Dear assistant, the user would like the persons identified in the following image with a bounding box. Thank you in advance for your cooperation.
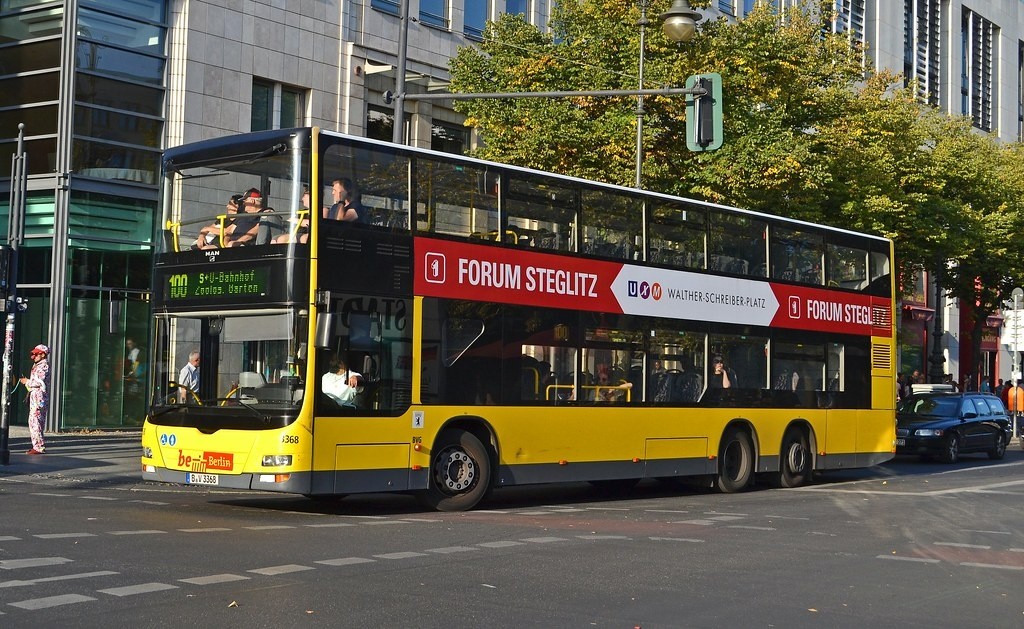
[520,351,734,404]
[895,370,1024,438]
[179,351,202,404]
[322,349,365,408]
[19,344,52,455]
[186,177,361,251]
[122,338,148,426]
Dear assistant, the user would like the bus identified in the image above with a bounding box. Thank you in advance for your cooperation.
[107,125,899,507]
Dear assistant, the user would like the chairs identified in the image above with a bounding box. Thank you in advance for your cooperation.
[551,365,839,403]
[350,351,372,375]
[206,206,822,284]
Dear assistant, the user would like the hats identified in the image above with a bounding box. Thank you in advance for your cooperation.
[238,188,261,200]
[31,344,49,354]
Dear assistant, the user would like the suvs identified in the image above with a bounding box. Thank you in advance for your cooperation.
[891,390,1014,463]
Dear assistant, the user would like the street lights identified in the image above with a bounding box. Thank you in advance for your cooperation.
[633,1,703,401]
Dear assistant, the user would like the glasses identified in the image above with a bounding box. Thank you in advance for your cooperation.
[713,359,723,363]
[32,352,42,356]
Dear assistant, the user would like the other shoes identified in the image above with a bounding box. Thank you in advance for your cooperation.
[26,450,41,454]
